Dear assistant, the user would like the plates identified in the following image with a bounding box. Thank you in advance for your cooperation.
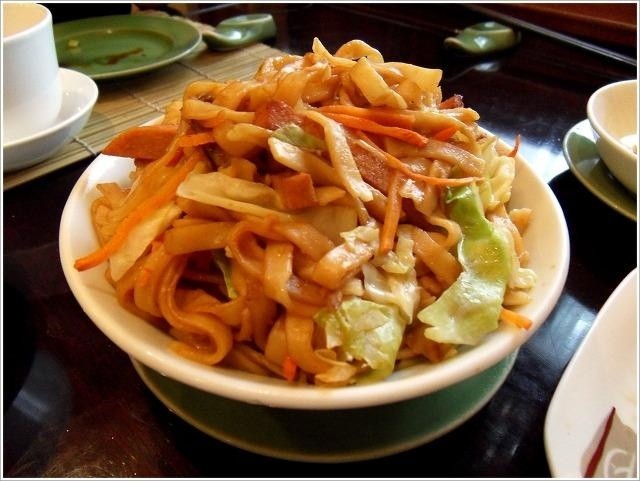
[128,344,523,461]
[562,118,637,224]
[544,265,637,478]
[53,15,203,78]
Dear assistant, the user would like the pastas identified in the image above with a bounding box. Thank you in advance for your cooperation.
[92,38,535,385]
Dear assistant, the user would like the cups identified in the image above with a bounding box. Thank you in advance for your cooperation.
[1,2,61,143]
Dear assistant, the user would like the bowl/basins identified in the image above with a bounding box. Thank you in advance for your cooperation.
[3,67,99,172]
[587,79,637,197]
[59,105,573,410]
